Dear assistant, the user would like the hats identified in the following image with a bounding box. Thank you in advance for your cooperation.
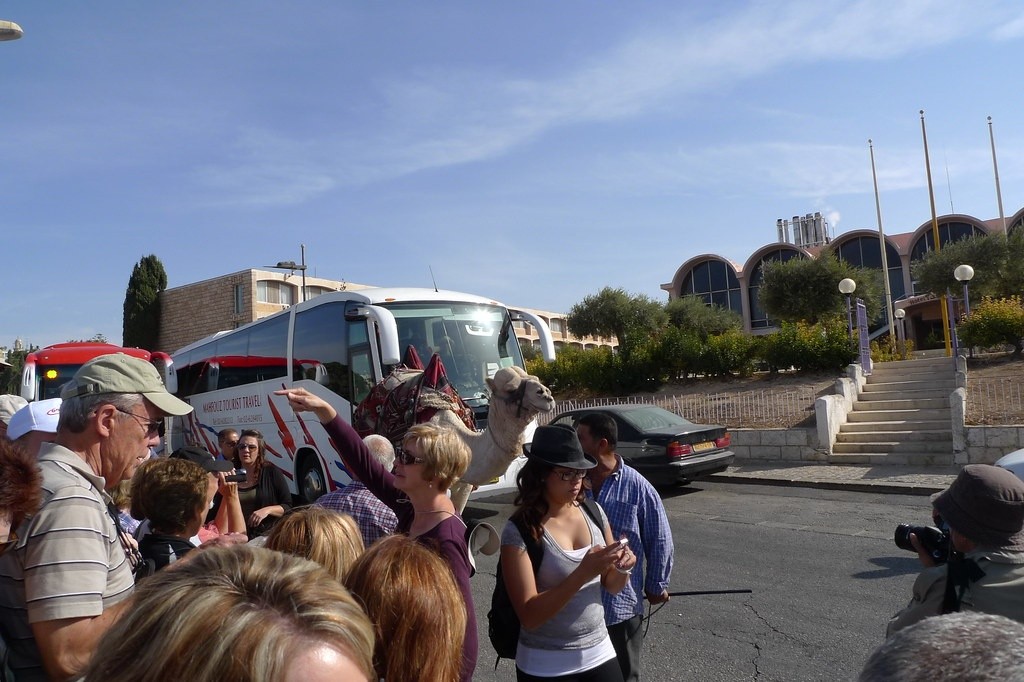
[0,394,29,425]
[7,398,62,441]
[523,423,598,469]
[465,519,501,578]
[61,353,194,417]
[931,464,1024,553]
[169,446,234,473]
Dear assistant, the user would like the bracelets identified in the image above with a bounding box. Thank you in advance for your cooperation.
[615,567,633,575]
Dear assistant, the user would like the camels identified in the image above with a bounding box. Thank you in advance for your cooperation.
[356,366,556,517]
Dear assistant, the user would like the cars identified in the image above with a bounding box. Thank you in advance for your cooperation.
[547,404,736,489]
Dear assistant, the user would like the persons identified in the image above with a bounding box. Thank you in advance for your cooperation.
[6,398,63,458]
[859,610,1024,682]
[108,447,248,575]
[501,424,636,682]
[0,394,29,425]
[886,464,1024,639]
[344,533,463,682]
[439,336,454,355]
[264,507,366,583]
[575,414,674,682]
[314,434,398,547]
[216,429,239,460]
[205,429,292,538]
[0,352,193,682]
[76,543,378,682]
[0,438,46,542]
[273,387,478,682]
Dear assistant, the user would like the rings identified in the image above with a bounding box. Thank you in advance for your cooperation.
[625,553,630,559]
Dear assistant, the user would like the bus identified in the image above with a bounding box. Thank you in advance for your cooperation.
[21,342,178,457]
[167,288,556,501]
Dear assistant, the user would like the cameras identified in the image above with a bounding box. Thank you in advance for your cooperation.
[895,524,960,567]
[219,470,247,485]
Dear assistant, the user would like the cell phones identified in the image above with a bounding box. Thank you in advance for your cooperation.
[607,539,629,556]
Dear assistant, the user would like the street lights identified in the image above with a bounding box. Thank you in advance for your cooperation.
[838,278,856,363]
[894,309,905,360]
[953,264,974,358]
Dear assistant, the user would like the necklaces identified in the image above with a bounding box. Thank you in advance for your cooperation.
[245,473,251,483]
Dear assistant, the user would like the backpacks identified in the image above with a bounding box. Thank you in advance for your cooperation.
[487,499,606,659]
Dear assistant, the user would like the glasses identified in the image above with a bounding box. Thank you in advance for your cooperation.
[239,444,258,452]
[94,407,166,437]
[395,447,426,465]
[552,470,587,480]
[0,532,20,557]
[225,441,238,446]
